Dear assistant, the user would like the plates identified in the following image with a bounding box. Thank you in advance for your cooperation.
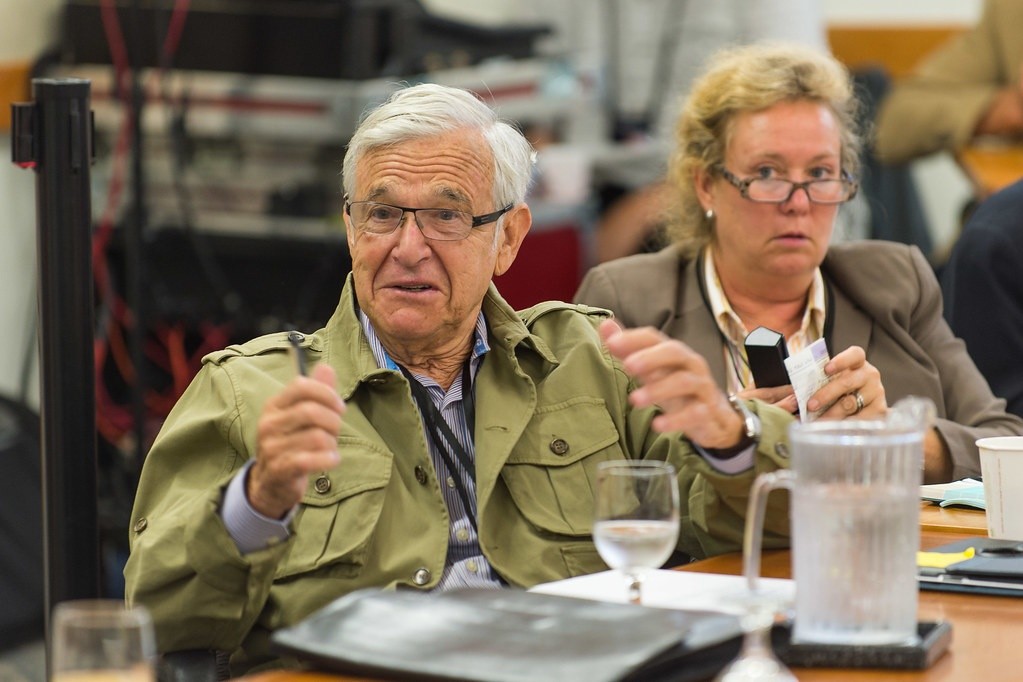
[525,568,798,639]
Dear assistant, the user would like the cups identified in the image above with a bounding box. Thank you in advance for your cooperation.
[977,437,1023,543]
[50,600,158,682]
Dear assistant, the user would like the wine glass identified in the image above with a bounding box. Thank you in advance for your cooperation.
[591,460,680,604]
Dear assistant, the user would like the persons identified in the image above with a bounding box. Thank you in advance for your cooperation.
[571,41,1022,484]
[870,0,1022,169]
[934,179,1023,418]
[125,85,802,676]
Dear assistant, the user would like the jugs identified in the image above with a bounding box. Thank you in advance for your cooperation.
[744,395,936,646]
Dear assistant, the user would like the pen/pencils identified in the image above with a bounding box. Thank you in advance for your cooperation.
[291,332,308,379]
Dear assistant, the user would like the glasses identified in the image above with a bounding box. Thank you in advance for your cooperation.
[343,193,512,242]
[713,162,858,204]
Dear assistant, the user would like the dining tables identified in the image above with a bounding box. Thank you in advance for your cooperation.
[222,496,1023,682]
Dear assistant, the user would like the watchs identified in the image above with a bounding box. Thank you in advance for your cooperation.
[702,392,763,461]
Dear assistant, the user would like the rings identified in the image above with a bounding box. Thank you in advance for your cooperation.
[855,392,864,413]
